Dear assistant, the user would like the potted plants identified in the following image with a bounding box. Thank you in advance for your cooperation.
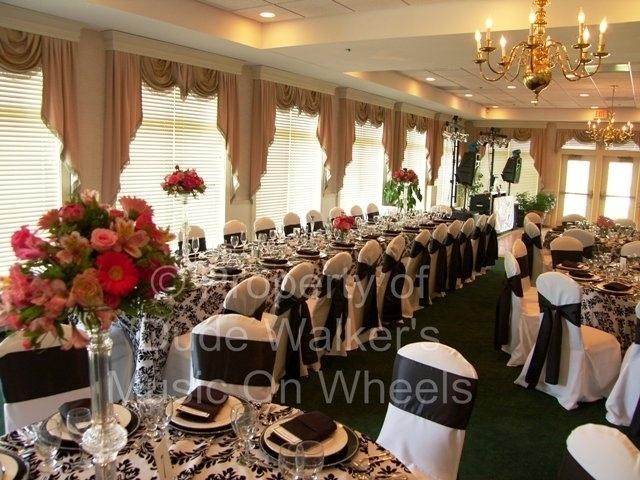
[516,191,557,227]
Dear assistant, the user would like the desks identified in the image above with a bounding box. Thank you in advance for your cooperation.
[0,391,415,480]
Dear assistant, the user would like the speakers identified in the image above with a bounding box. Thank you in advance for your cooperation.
[501,156,522,184]
[456,151,480,187]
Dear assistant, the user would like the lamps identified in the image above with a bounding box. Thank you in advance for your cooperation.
[474,0,611,106]
[586,85,635,151]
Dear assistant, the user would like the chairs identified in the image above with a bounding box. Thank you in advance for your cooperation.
[115,203,638,417]
[375,342,478,477]
[3,323,94,435]
[554,426,636,479]
[186,315,278,404]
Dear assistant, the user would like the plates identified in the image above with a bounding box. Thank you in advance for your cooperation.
[45,401,130,441]
[0,452,18,479]
[262,418,349,457]
[596,282,633,294]
[258,425,357,466]
[0,448,30,480]
[567,271,601,280]
[170,396,247,428]
[166,397,252,436]
[36,409,141,451]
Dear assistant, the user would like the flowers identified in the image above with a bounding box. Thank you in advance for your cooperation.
[158,163,207,201]
[391,167,420,187]
[0,187,183,352]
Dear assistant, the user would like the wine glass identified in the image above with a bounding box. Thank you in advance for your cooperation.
[33,419,62,473]
[137,390,172,442]
[278,440,324,480]
[191,204,452,267]
[574,219,640,284]
[231,403,258,467]
[66,409,91,468]
